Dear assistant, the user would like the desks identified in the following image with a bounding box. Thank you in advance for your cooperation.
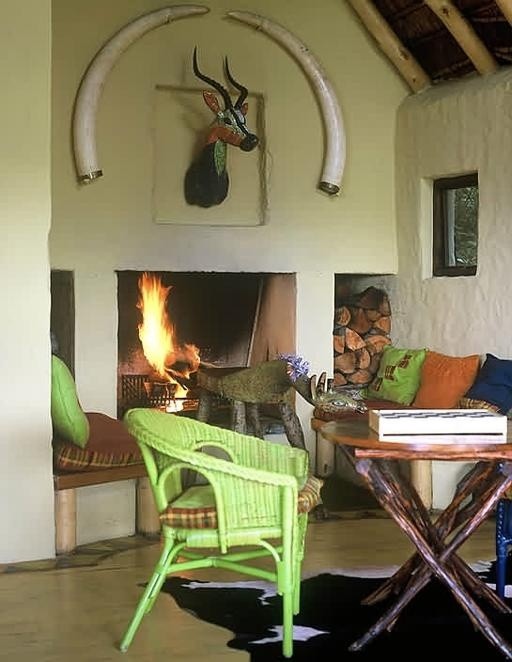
[310,417,511,661]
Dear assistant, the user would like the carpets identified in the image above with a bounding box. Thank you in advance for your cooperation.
[133,556,512,662]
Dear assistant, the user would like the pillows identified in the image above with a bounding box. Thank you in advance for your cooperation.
[413,350,483,407]
[50,408,140,471]
[466,352,512,412]
[366,348,428,406]
[52,354,92,448]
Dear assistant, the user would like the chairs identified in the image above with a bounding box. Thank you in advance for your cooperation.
[114,406,325,657]
